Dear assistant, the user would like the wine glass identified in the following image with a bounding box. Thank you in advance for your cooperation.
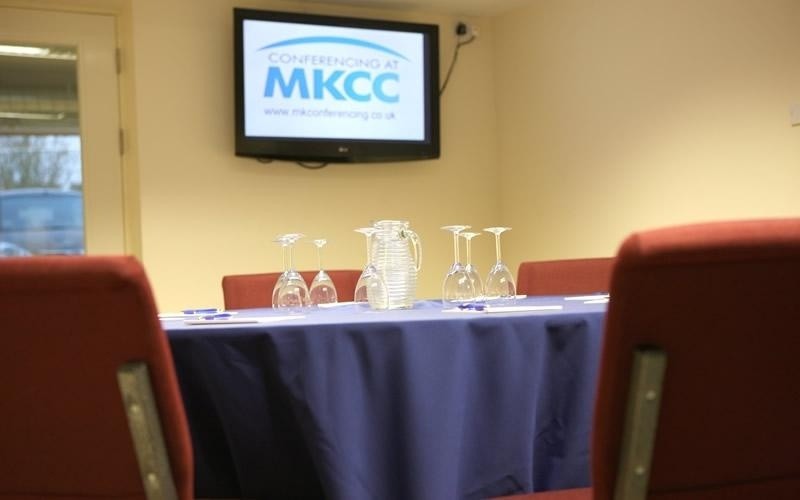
[441,226,516,309]
[272,233,338,315]
[353,228,390,311]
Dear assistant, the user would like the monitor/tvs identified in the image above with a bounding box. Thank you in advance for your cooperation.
[233,7,439,162]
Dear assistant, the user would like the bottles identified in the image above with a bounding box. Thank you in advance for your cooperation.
[366,221,422,309]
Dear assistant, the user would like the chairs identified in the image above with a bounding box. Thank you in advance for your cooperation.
[221,269,367,310]
[483,218,800,500]
[513,257,620,296]
[0,252,195,500]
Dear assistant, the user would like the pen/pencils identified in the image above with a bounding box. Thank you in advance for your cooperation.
[184,308,233,322]
[456,302,491,310]
